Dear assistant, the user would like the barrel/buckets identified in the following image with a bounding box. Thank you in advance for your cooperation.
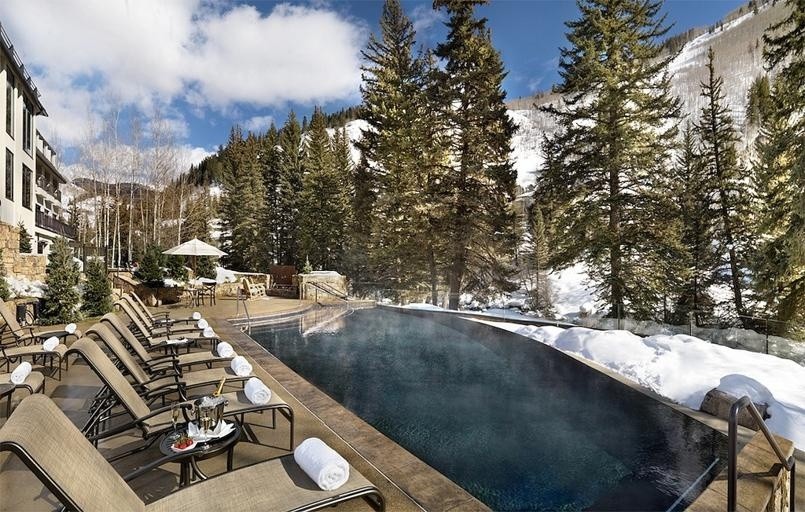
[193,396,228,428]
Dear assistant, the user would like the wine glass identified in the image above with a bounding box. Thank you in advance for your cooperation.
[200,417,213,450]
[170,402,180,439]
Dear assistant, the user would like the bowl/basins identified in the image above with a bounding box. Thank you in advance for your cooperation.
[172,440,197,452]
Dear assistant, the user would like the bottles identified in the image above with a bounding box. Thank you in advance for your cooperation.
[211,377,225,400]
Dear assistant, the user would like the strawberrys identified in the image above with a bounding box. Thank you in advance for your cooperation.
[174,435,193,448]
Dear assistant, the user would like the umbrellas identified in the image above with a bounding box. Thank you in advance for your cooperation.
[159,234,229,280]
